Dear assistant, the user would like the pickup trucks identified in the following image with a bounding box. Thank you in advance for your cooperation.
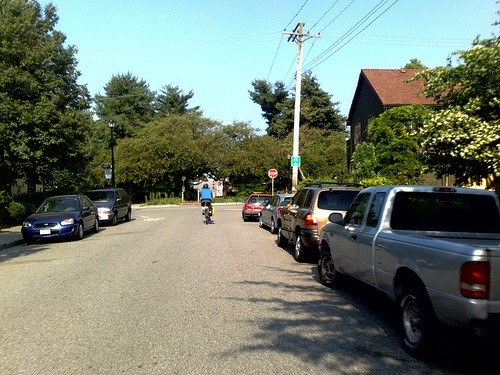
[319,184,500,357]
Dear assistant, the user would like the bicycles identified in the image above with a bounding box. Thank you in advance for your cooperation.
[200,200,213,224]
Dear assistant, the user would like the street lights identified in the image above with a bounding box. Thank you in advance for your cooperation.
[102,119,116,188]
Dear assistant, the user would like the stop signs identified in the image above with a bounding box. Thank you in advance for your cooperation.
[268,169,278,179]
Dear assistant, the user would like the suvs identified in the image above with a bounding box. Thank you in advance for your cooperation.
[83,188,132,224]
[258,184,368,262]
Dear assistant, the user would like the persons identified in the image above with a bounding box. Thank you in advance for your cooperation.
[199,183,213,216]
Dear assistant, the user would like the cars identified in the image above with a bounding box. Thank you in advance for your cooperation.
[21,195,99,242]
[242,193,273,221]
